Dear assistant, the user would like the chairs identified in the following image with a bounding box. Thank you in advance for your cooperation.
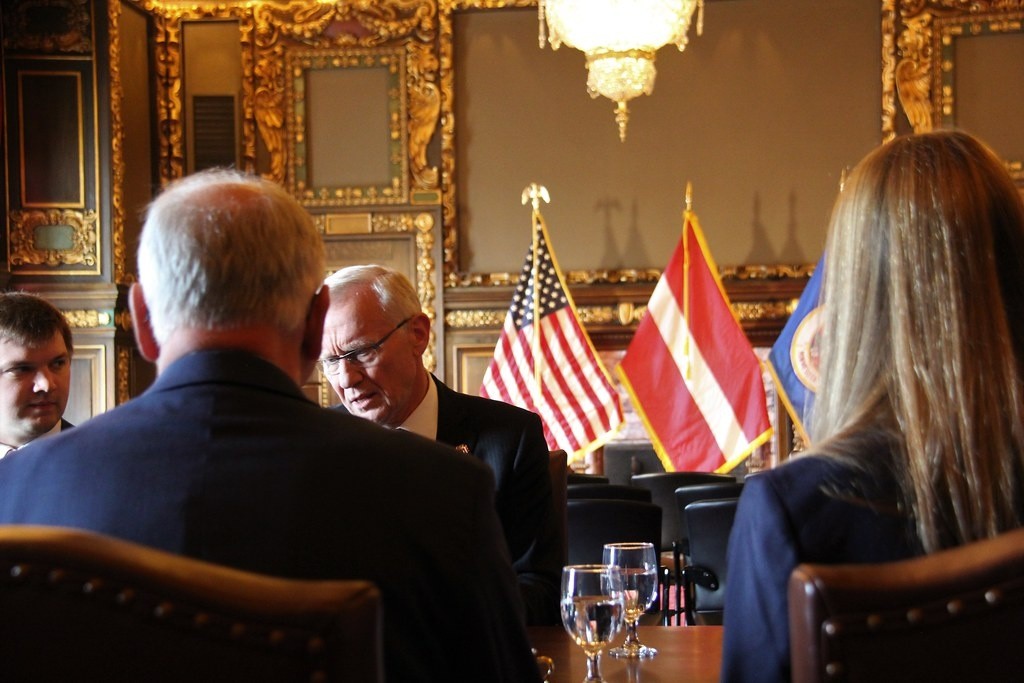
[547,448,746,623]
[786,533,1024,683]
[0,524,383,683]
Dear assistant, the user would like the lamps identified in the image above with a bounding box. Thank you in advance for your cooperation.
[537,0,704,144]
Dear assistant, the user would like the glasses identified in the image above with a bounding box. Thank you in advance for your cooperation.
[315,316,413,375]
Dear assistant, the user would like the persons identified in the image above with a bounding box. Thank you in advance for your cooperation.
[314,262,575,619]
[0,289,79,456]
[1,167,535,683]
[716,128,1023,680]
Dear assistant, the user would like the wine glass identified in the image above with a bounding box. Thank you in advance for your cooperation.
[600,543,659,656]
[561,564,626,683]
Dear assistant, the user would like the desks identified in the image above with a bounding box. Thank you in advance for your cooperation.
[527,626,723,683]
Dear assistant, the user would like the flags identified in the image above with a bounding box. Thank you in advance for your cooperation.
[768,247,831,440]
[477,213,629,463]
[617,207,774,473]
